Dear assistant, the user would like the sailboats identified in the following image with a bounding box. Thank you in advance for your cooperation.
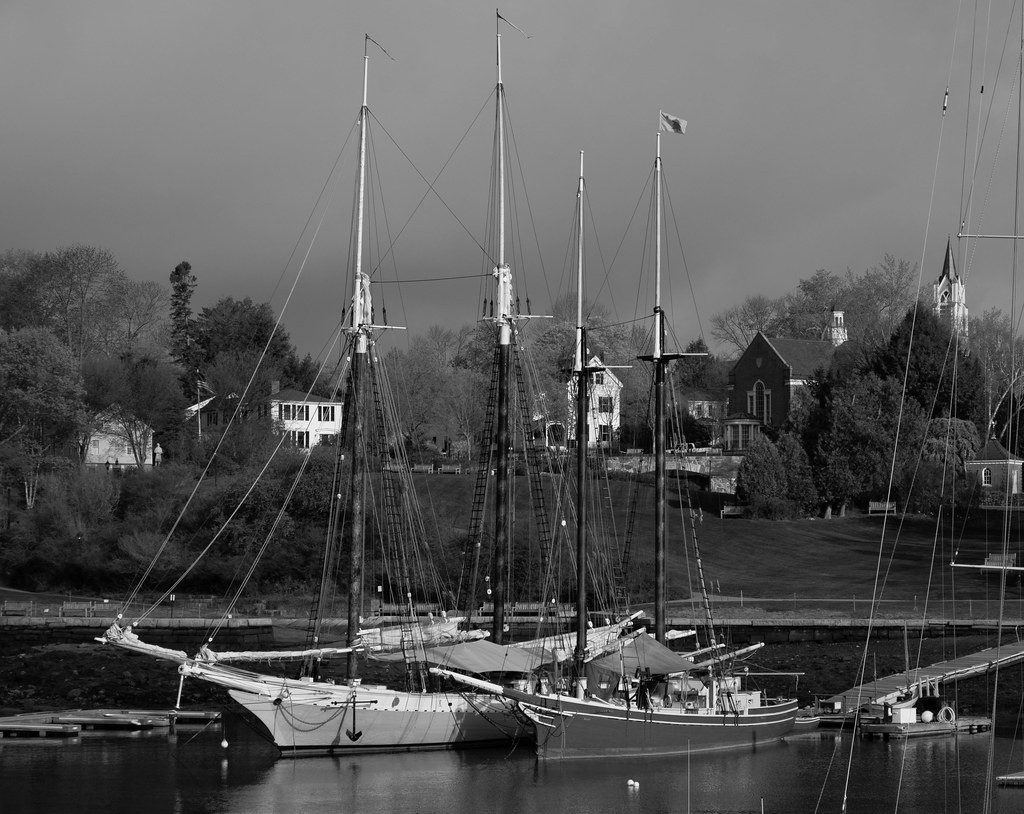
[92,7,823,767]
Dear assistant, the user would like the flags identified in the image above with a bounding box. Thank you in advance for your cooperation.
[659,111,688,135]
[197,372,217,394]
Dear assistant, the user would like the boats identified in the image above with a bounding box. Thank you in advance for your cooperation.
[856,677,994,742]
